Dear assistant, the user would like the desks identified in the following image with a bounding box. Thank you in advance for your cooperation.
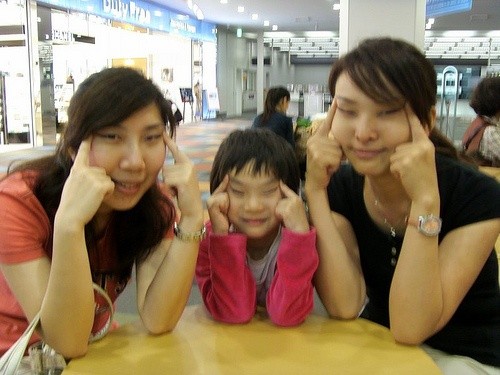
[61,304,442,375]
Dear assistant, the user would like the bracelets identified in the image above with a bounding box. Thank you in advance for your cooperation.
[173,222,207,242]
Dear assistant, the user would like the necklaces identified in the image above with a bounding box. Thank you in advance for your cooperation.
[369,188,412,238]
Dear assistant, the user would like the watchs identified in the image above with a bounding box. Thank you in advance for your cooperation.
[402,212,443,237]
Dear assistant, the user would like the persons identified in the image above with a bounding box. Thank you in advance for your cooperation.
[304,36,500,375]
[0,66,205,360]
[252,85,307,197]
[460,74,500,168]
[193,127,319,327]
